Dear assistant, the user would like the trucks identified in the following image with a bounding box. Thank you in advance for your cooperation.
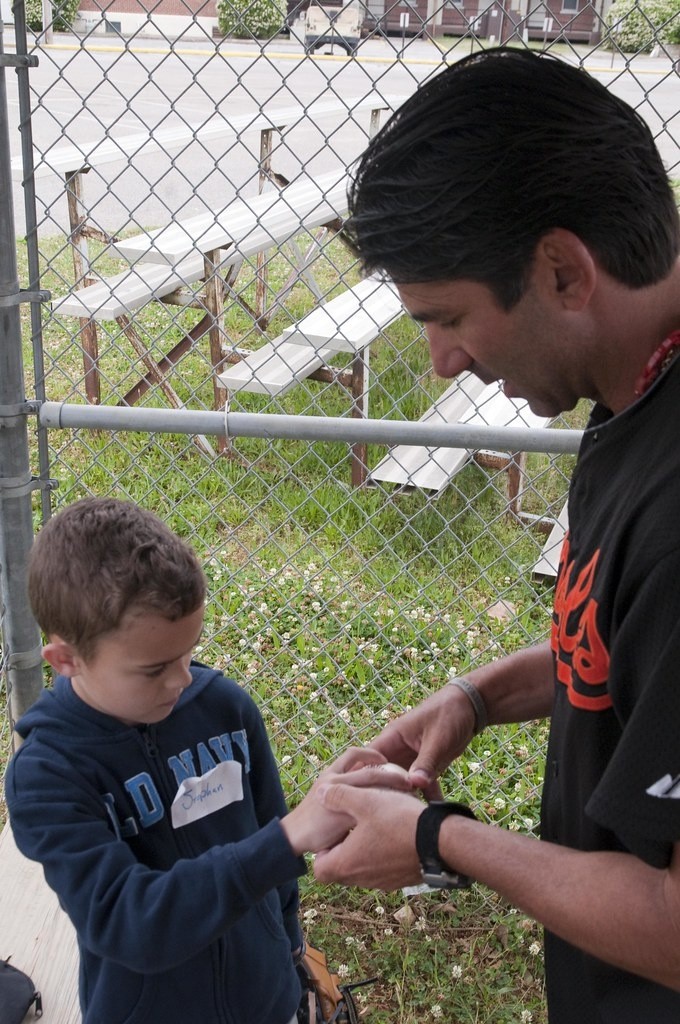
[302,5,362,58]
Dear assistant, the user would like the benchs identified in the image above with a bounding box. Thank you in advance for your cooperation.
[49,204,363,322]
[527,492,570,589]
[366,369,498,500]
[215,334,340,397]
[278,268,407,354]
[458,376,552,430]
[111,169,363,267]
[8,91,413,175]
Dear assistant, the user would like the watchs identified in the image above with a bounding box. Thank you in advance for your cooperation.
[413,799,479,890]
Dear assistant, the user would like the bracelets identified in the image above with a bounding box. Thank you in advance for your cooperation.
[447,676,487,735]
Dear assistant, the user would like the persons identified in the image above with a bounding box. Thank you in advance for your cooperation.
[311,46,680,1024]
[6,494,414,1024]
